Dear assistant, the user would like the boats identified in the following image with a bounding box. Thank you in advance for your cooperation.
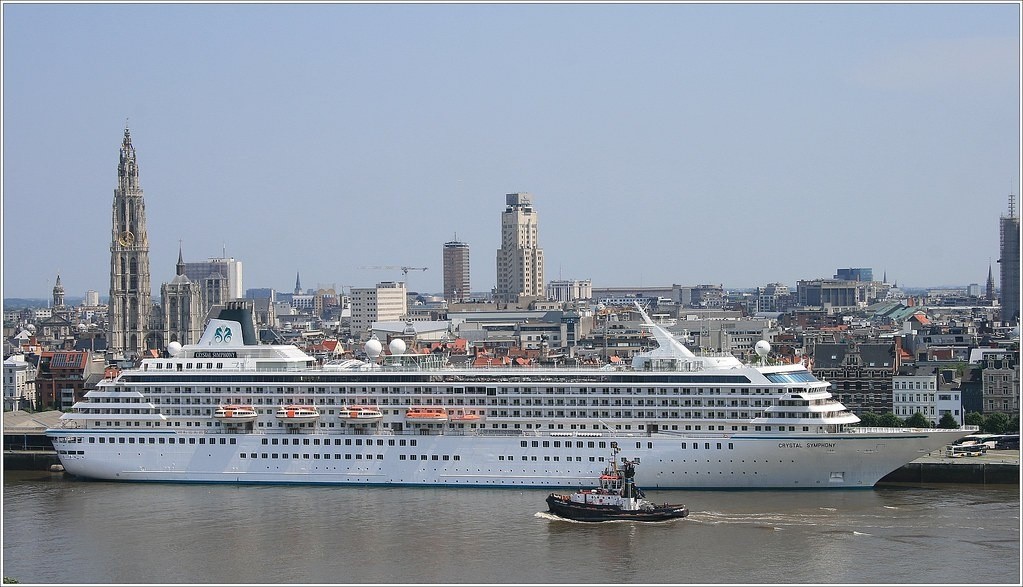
[543,439,690,522]
[449,414,480,425]
[403,407,449,424]
[274,405,320,424]
[213,406,258,424]
[338,405,383,425]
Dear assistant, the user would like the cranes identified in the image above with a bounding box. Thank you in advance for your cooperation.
[356,265,429,287]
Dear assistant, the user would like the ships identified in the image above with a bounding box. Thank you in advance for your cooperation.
[41,292,981,490]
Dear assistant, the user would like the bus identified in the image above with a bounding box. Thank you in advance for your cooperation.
[952,433,997,450]
[946,444,991,458]
